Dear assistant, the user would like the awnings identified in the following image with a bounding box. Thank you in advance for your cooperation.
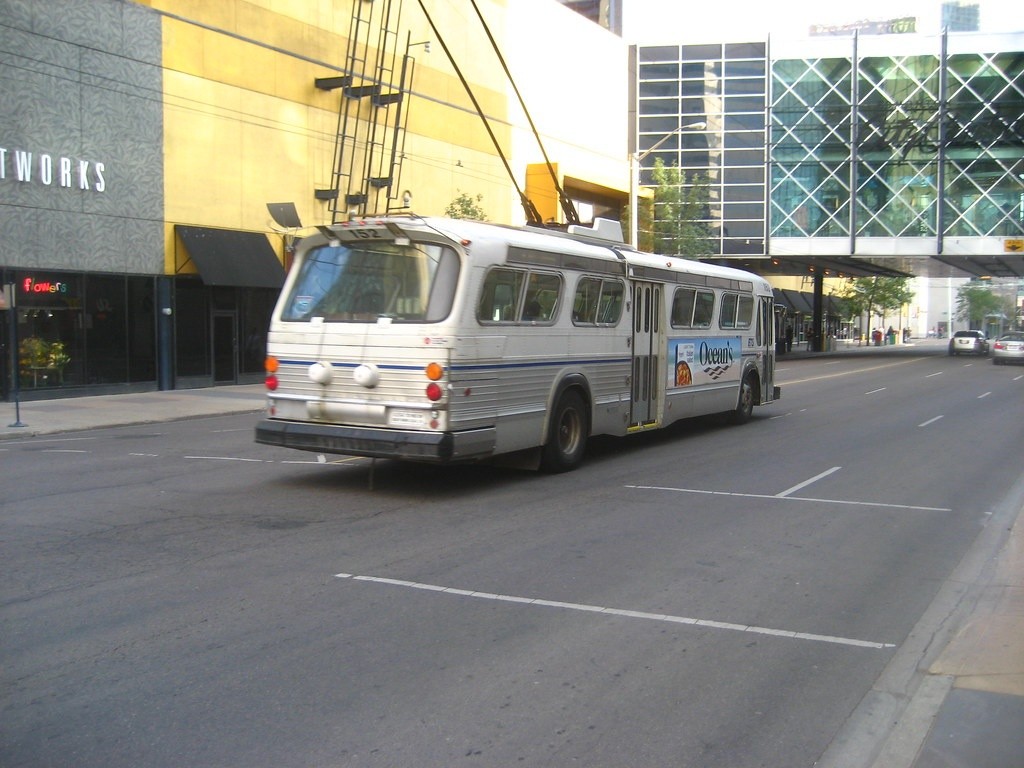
[174,225,287,288]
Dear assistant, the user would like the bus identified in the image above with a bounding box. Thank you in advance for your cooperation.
[256,1,782,482]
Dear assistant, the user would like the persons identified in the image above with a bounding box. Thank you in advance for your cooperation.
[872,327,877,342]
[807,328,814,351]
[529,301,543,317]
[905,326,912,341]
[887,326,893,337]
[785,325,793,352]
[937,325,945,341]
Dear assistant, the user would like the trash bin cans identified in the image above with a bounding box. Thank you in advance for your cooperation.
[874,331,882,346]
[884,330,899,344]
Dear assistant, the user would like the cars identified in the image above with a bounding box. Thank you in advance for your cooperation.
[992,332,1024,366]
[949,330,991,356]
